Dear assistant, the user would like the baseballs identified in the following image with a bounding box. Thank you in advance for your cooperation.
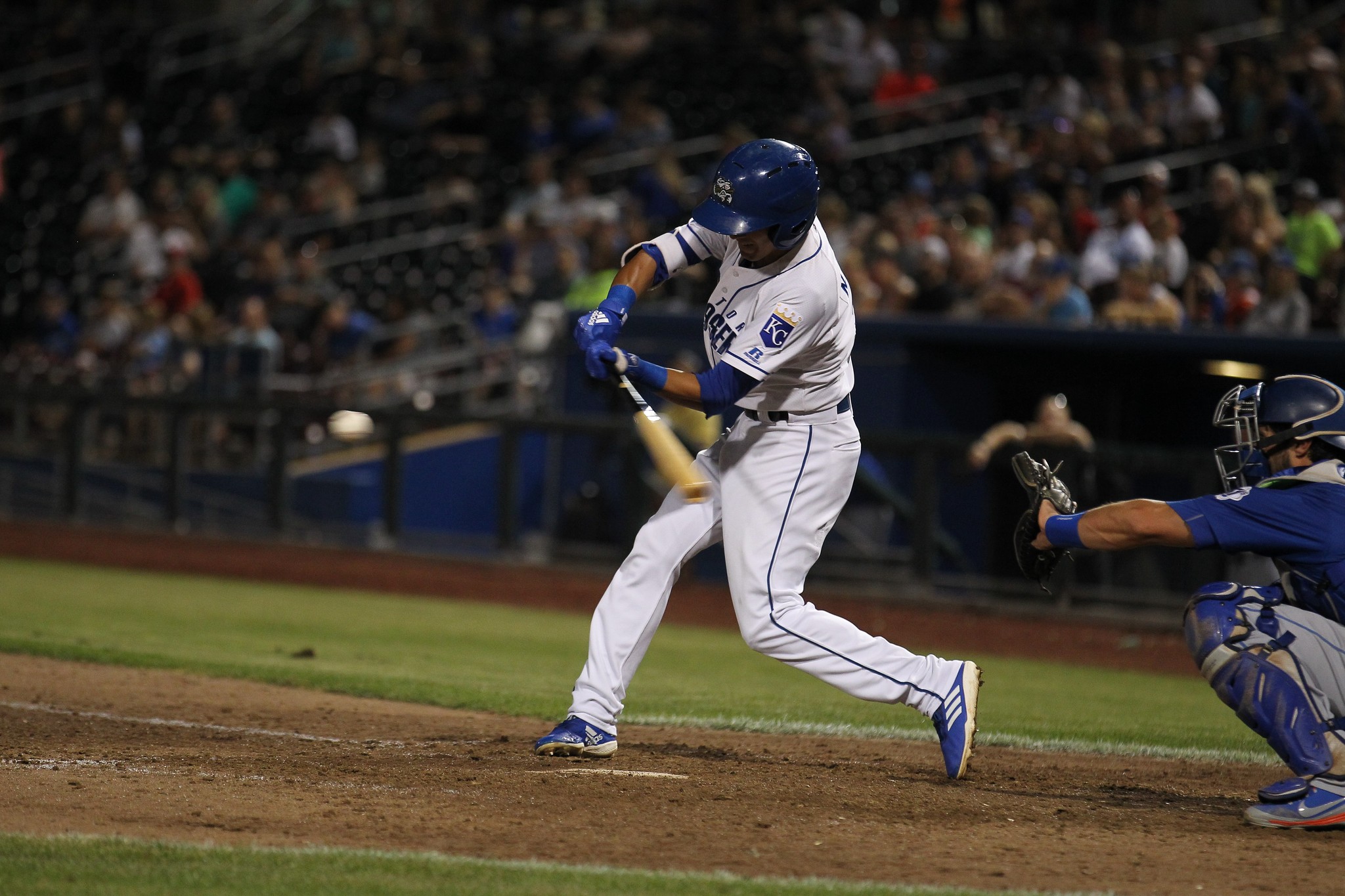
[327,410,374,442]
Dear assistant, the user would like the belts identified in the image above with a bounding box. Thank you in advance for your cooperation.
[736,395,852,424]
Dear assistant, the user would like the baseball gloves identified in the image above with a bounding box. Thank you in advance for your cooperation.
[1012,451,1074,597]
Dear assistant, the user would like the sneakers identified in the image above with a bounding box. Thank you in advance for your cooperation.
[534,710,621,766]
[1257,773,1322,807]
[926,658,980,785]
[1243,770,1345,838]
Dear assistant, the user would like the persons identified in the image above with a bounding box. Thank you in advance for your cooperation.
[531,138,985,779]
[1014,376,1345,830]
[3,1,1343,374]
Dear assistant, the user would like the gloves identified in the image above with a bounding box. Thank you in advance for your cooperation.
[572,282,643,385]
[591,334,667,393]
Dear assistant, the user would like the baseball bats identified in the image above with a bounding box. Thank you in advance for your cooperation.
[612,371,712,505]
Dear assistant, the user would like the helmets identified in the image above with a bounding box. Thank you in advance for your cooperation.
[1255,370,1345,469]
[688,136,821,241]
